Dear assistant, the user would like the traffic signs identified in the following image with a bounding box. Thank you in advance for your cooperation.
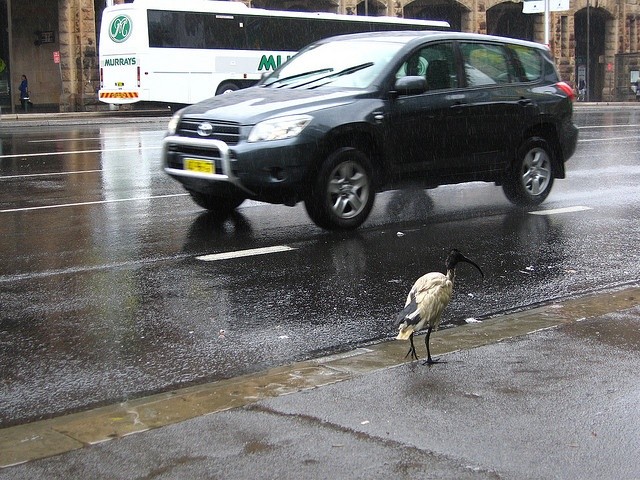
[522,1,570,12]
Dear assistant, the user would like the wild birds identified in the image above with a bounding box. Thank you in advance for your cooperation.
[392,249,484,367]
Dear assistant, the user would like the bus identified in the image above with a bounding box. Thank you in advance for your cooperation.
[97,0,452,107]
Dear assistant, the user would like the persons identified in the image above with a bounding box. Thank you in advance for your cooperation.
[19,73,31,111]
[577,76,587,101]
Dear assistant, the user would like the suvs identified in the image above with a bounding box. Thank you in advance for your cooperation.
[164,30,581,233]
